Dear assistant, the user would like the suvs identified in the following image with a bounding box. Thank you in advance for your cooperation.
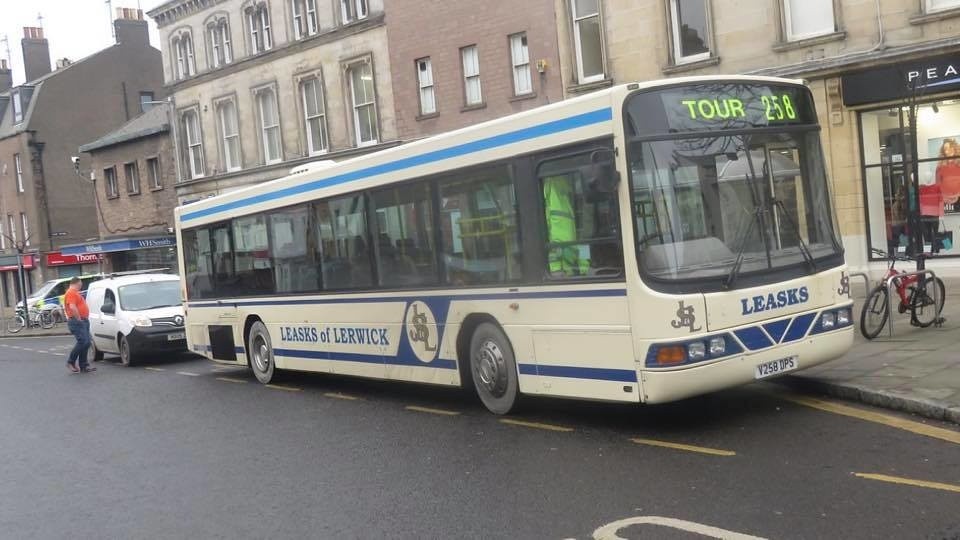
[83,272,203,369]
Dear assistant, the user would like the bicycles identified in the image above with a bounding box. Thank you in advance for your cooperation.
[6,303,58,334]
[858,247,946,340]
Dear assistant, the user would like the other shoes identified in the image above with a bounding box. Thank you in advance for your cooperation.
[68,362,79,373]
[81,367,96,374]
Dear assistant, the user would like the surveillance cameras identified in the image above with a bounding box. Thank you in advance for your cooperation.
[71,157,80,164]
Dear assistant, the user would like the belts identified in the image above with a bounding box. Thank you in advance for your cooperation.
[69,316,88,320]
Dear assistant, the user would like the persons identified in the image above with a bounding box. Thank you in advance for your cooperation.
[589,202,619,276]
[919,171,944,253]
[935,138,960,212]
[64,277,97,372]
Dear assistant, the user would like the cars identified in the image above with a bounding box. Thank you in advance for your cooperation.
[12,272,116,324]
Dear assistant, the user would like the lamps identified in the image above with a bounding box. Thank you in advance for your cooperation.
[888,108,897,117]
[932,102,938,112]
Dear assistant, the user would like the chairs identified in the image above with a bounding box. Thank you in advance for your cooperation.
[321,233,463,291]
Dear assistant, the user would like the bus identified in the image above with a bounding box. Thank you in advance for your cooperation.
[169,73,857,417]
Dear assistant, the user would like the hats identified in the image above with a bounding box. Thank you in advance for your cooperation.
[70,277,80,284]
